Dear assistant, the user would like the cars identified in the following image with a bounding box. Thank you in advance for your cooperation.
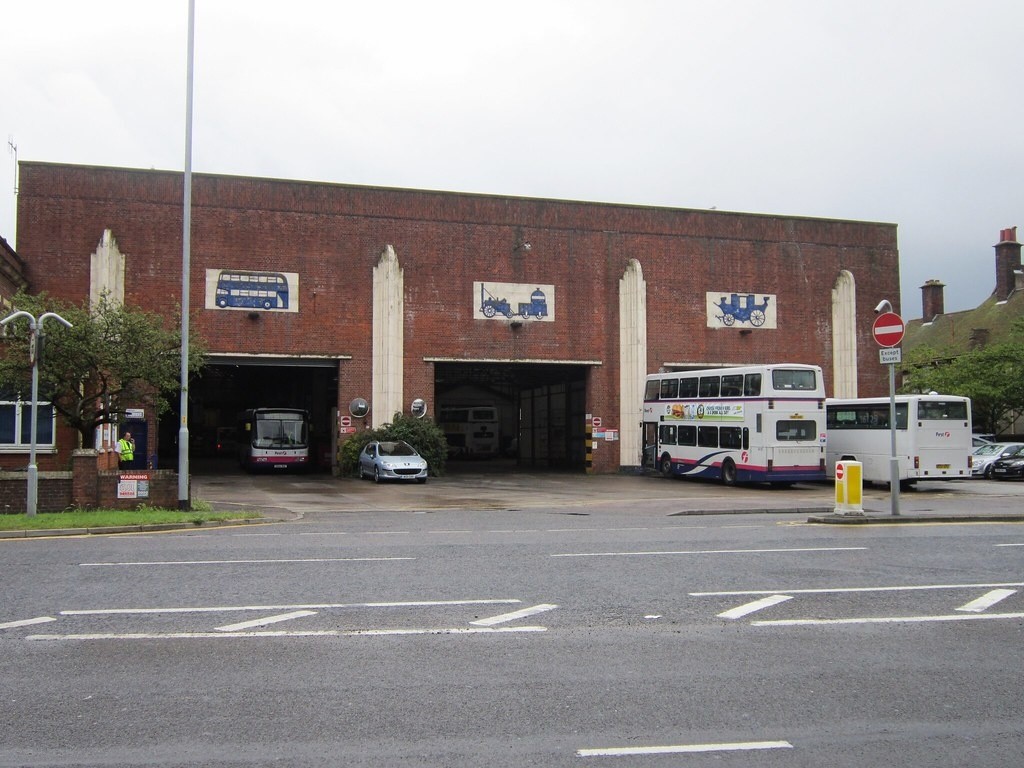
[972,437,1024,479]
[992,446,1024,481]
[358,440,428,484]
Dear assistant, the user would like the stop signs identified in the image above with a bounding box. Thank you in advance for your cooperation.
[592,417,601,427]
[872,311,905,347]
[837,464,844,479]
[341,416,351,426]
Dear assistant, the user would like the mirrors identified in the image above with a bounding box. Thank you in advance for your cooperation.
[410,399,427,418]
[348,398,369,418]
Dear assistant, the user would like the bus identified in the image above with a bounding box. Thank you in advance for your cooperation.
[239,408,313,474]
[639,363,828,485]
[440,406,499,458]
[825,394,973,486]
[211,427,239,455]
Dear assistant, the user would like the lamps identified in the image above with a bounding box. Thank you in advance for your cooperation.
[247,312,260,319]
[739,329,752,336]
[514,242,532,253]
[511,322,523,328]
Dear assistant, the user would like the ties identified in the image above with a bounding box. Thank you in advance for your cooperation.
[126,442,130,449]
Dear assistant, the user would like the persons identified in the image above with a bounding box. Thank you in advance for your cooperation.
[114,433,136,470]
[288,427,301,444]
[367,444,371,448]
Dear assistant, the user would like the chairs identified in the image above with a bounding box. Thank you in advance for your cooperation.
[927,409,938,419]
[708,390,718,397]
[670,391,697,397]
[919,410,925,418]
[729,388,740,396]
